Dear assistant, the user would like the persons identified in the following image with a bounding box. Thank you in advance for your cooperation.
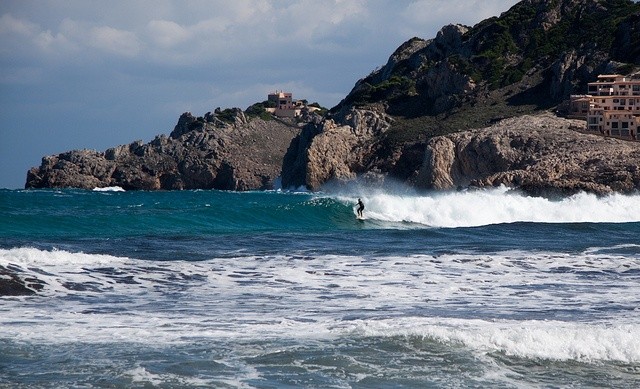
[355,198,365,219]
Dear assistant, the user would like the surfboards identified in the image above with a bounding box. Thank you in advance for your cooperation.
[356,216,365,220]
